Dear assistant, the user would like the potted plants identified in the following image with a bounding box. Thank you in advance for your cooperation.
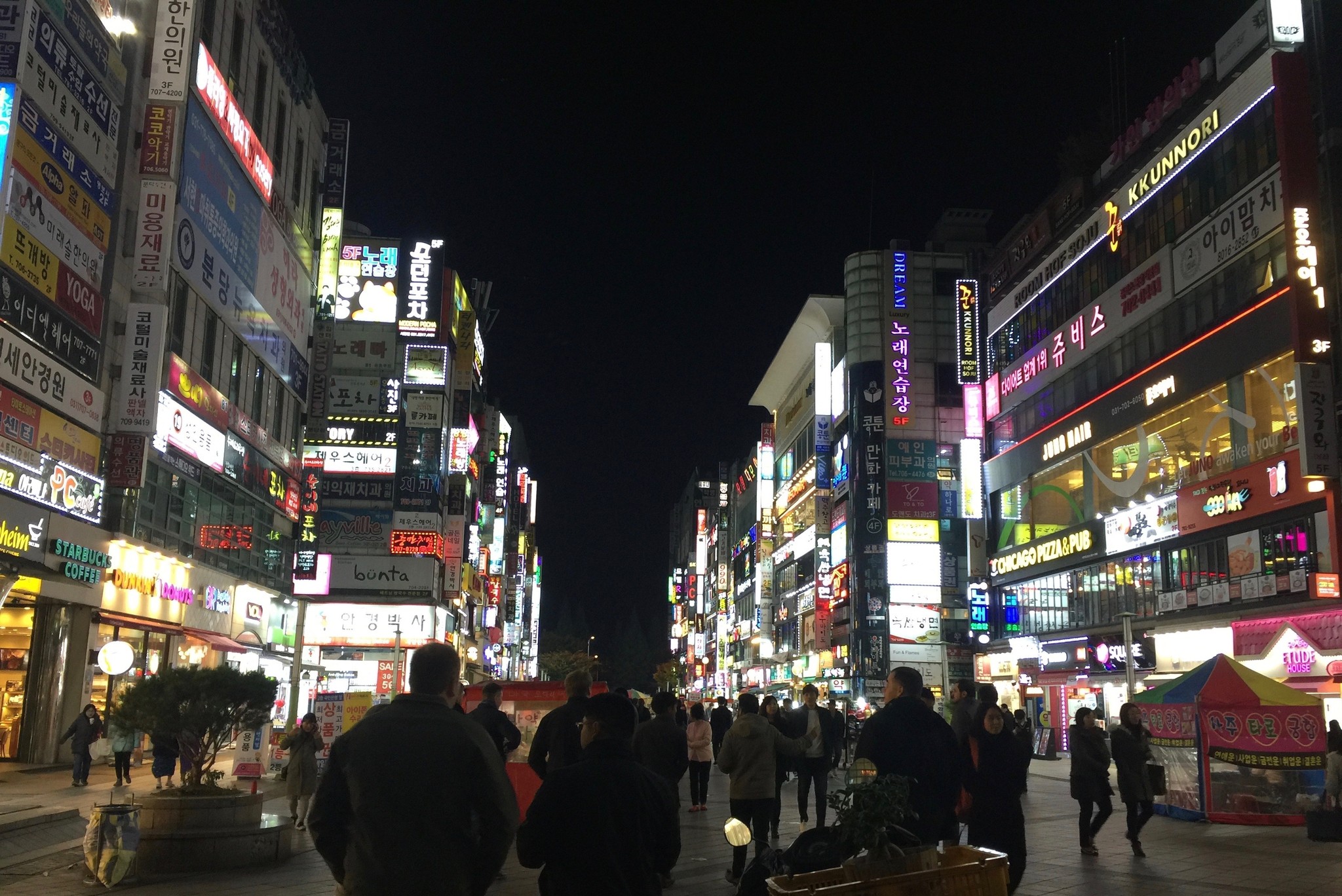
[764,773,1010,896]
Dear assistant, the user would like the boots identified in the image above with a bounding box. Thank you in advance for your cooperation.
[165,775,175,787]
[156,777,162,788]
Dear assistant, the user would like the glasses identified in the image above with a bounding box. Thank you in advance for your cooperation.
[575,719,603,729]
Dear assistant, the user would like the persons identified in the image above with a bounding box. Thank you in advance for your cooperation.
[1106,703,1155,856]
[854,666,960,844]
[1328,720,1341,738]
[716,683,845,885]
[309,642,521,896]
[1326,730,1342,807]
[1069,707,1114,854]
[686,703,712,812]
[706,703,714,721]
[711,696,736,764]
[960,702,1027,896]
[515,670,689,896]
[950,679,998,745]
[280,713,325,830]
[112,717,140,787]
[1011,709,1035,793]
[152,715,200,787]
[59,704,103,785]
[1001,703,1014,718]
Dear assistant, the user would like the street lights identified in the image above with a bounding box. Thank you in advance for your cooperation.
[936,641,950,704]
[658,686,661,693]
[728,665,735,699]
[665,679,671,692]
[706,671,712,698]
[588,636,594,672]
[676,678,682,699]
[1114,611,1139,701]
[759,657,769,698]
[690,674,694,695]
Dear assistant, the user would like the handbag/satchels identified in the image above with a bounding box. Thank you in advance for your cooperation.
[957,737,979,813]
[1305,787,1342,843]
[281,766,287,779]
[1145,757,1168,796]
[88,733,111,759]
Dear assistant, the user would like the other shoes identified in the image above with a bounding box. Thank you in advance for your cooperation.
[700,804,708,811]
[831,766,838,776]
[658,873,676,888]
[113,779,123,786]
[725,868,739,886]
[827,771,835,778]
[689,805,699,812]
[180,774,186,786]
[123,774,131,784]
[296,821,306,830]
[81,779,87,785]
[1080,837,1100,855]
[771,826,779,840]
[496,871,507,880]
[800,819,808,833]
[72,781,78,786]
[1125,831,1145,857]
[291,813,298,824]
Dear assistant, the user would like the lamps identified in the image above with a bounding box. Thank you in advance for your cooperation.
[302,669,310,679]
[1308,480,1325,493]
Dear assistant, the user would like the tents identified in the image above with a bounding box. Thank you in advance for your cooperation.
[1134,653,1328,825]
